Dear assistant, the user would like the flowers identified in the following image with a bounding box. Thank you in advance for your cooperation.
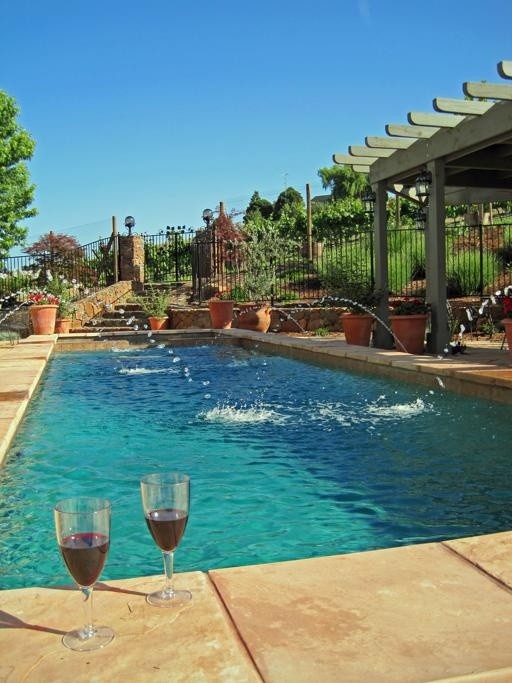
[388,296,433,316]
[29,293,59,305]
[503,296,512,318]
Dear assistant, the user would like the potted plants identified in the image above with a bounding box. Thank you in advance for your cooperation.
[131,224,387,347]
[54,288,78,334]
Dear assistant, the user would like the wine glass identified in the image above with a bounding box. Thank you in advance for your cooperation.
[53,496,113,652]
[140,470,192,607]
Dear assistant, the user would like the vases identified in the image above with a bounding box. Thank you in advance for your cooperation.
[29,304,60,335]
[500,318,512,353]
[388,314,429,355]
